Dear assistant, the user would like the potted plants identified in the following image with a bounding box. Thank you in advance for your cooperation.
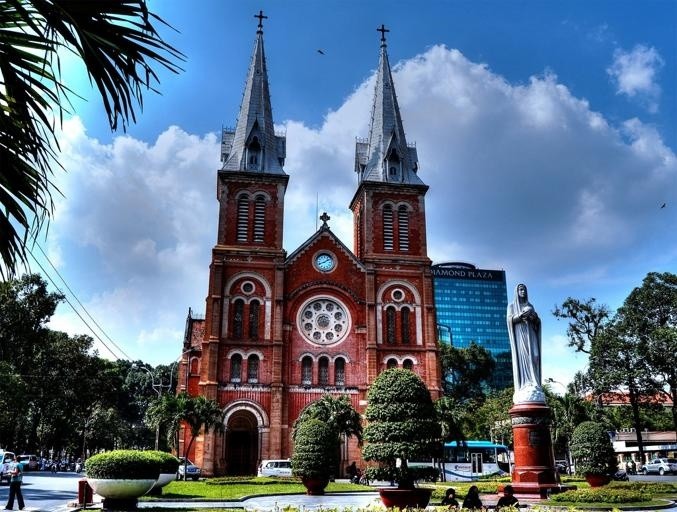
[289,418,339,497]
[84,447,182,512]
[360,364,445,508]
[567,420,620,488]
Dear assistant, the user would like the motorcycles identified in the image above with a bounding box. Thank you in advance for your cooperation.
[351,469,368,485]
[45,460,82,473]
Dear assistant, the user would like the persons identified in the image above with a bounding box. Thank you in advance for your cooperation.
[495,485,520,512]
[630,460,636,471]
[349,461,369,486]
[462,485,486,510]
[442,488,460,512]
[3,455,26,511]
[506,283,542,391]
[39,456,83,470]
[625,460,629,475]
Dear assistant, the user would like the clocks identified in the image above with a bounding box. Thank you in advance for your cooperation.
[315,250,335,272]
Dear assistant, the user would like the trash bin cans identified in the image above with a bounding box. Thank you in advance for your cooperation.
[79,480,93,503]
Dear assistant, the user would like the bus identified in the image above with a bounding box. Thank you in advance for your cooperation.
[394,440,513,486]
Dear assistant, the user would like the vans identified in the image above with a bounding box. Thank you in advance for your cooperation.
[17,455,38,471]
[257,460,296,477]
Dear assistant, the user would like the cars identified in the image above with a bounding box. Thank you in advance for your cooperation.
[176,457,201,480]
[555,460,584,475]
[643,458,677,475]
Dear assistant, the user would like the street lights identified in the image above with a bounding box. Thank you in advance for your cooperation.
[131,346,195,450]
[548,378,576,476]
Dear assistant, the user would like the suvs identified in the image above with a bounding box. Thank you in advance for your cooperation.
[0,451,17,484]
